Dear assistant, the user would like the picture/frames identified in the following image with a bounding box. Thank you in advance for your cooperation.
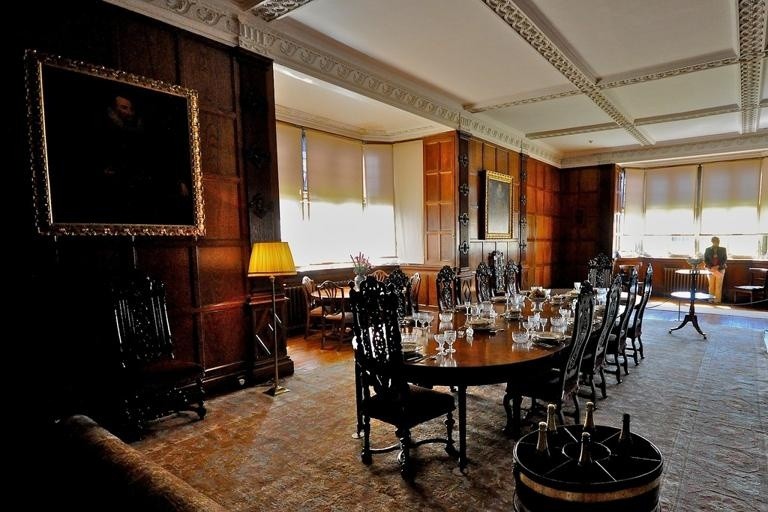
[484,168,514,239]
[23,48,207,237]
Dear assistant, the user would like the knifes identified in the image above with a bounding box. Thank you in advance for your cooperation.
[407,352,436,363]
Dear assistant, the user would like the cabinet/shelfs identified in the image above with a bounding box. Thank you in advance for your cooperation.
[669,252,716,340]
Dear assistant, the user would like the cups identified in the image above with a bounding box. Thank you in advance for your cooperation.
[469,307,478,316]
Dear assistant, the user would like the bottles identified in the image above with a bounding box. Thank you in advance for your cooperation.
[577,432,593,483]
[534,422,551,465]
[582,402,596,445]
[617,414,632,457]
[546,404,558,443]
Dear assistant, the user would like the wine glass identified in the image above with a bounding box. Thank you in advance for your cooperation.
[434,334,445,351]
[436,352,456,367]
[559,309,567,321]
[444,331,456,353]
[464,302,470,316]
[412,312,433,329]
[574,282,580,292]
[522,315,547,338]
[504,293,510,304]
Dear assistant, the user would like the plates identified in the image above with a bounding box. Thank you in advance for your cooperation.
[549,302,563,306]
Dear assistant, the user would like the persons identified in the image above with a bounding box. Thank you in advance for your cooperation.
[703,236,727,304]
[101,94,191,224]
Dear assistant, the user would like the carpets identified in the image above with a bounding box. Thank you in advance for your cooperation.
[83,308,768,511]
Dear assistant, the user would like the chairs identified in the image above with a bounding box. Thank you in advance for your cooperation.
[105,269,208,439]
[734,268,767,306]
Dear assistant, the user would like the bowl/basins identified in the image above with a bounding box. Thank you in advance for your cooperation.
[530,287,542,292]
[439,314,452,322]
[511,332,528,344]
[542,289,550,296]
[551,297,561,303]
[550,317,563,326]
[438,322,452,331]
[511,343,528,353]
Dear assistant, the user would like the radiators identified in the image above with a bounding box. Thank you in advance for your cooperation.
[664,267,710,293]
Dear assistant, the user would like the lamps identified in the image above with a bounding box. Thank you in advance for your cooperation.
[246,240,298,396]
